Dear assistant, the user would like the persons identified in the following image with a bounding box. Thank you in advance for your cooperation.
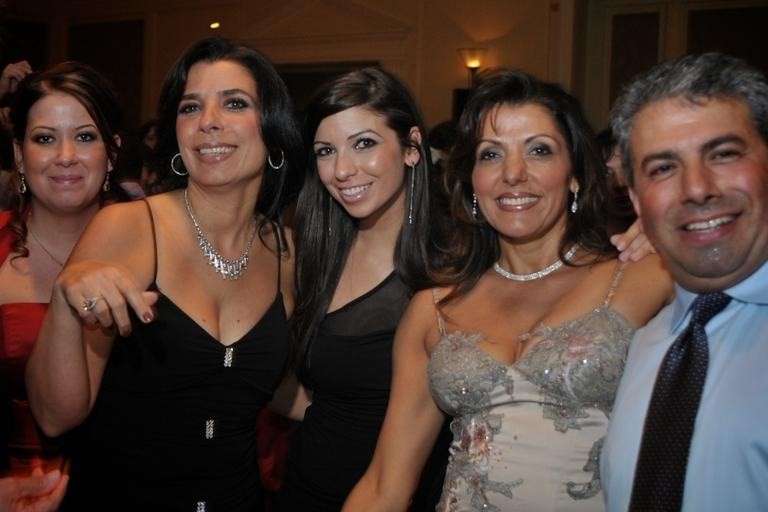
[0,466,69,512]
[136,118,165,149]
[2,60,36,211]
[22,34,297,512]
[2,59,150,512]
[114,171,147,201]
[594,51,768,512]
[583,116,637,256]
[289,66,657,512]
[337,65,676,512]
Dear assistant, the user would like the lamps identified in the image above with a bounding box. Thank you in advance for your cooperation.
[457,47,488,80]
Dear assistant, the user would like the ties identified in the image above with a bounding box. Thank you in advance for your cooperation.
[626,289,730,510]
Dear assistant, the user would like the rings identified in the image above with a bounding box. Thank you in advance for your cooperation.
[82,294,104,311]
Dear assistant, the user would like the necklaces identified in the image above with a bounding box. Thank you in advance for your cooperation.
[486,240,586,283]
[185,188,261,281]
[25,218,63,268]
